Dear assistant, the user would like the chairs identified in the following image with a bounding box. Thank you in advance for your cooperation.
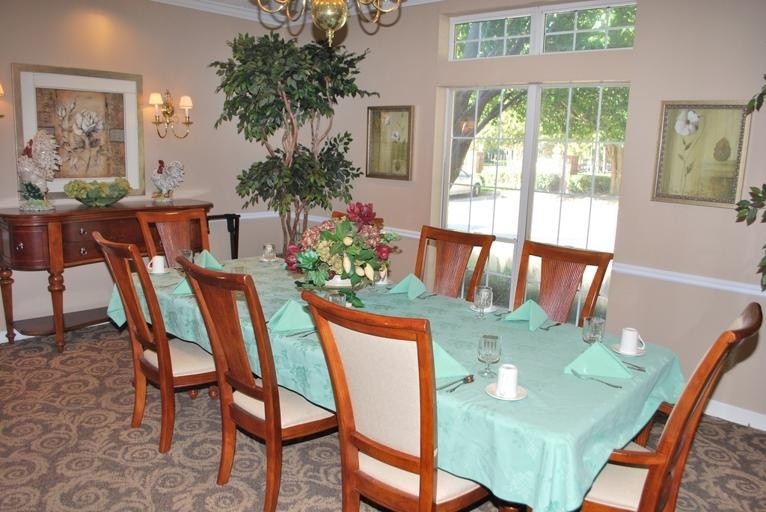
[176,255,337,512]
[512,239,614,329]
[136,208,210,399]
[301,291,491,512]
[576,303,764,512]
[90,230,218,453]
[415,225,496,302]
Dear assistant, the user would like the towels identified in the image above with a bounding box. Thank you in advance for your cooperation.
[502,300,549,332]
[194,250,223,270]
[171,276,194,296]
[267,299,315,333]
[431,341,471,378]
[388,272,425,301]
[566,342,634,379]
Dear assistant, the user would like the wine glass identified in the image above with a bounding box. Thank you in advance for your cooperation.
[477,334,502,379]
[472,285,493,320]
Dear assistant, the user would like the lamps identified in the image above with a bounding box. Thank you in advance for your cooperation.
[148,88,195,139]
[256,1,409,49]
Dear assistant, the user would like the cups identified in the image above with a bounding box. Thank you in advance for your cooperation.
[496,363,519,398]
[147,255,165,273]
[620,326,645,352]
[582,315,606,344]
[176,248,193,268]
[262,243,276,260]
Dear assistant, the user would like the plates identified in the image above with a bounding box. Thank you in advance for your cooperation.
[149,271,170,276]
[76,197,121,206]
[613,343,646,357]
[470,304,497,313]
[485,383,529,401]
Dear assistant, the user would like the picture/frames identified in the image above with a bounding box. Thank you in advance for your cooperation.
[366,106,414,181]
[9,62,146,195]
[651,99,753,209]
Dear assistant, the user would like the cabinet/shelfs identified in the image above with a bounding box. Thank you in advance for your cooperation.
[0,198,214,352]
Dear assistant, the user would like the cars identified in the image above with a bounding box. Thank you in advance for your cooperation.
[449,168,484,197]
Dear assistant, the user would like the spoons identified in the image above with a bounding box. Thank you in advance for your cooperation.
[539,322,561,330]
[436,375,475,393]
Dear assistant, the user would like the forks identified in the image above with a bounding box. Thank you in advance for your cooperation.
[570,369,623,389]
